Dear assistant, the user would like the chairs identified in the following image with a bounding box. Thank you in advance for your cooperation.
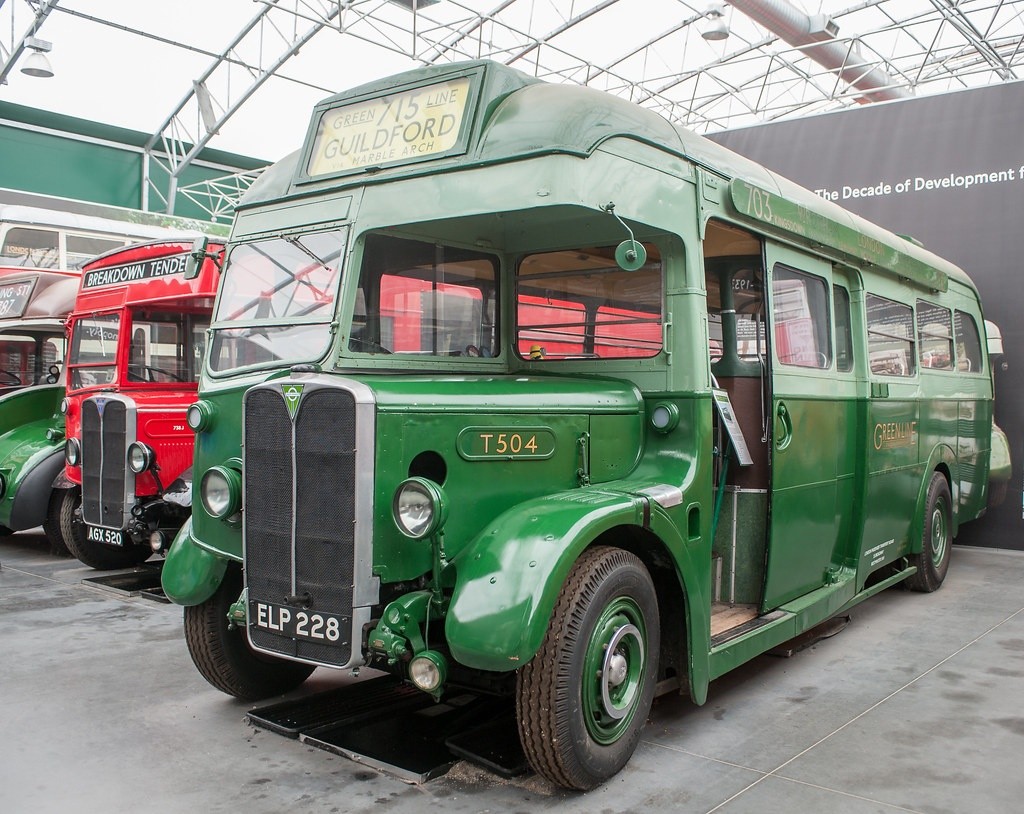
[871,361,906,375]
[942,358,971,371]
[791,351,827,369]
[921,352,933,368]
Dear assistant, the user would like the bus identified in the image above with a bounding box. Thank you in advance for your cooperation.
[159,58,1013,795]
[0,272,208,557]
[51,237,721,571]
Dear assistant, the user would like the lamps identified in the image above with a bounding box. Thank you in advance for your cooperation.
[701,3,729,41]
[20,36,54,78]
